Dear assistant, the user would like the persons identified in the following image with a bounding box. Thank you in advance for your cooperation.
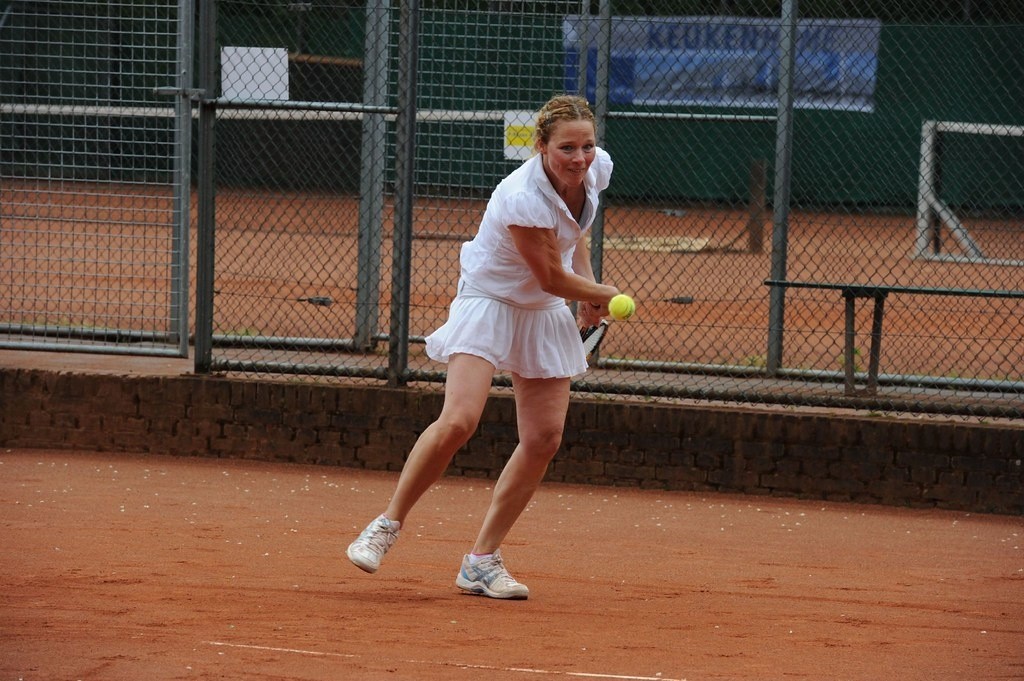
[345,94,620,599]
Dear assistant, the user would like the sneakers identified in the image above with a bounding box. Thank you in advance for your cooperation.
[454,550,529,600]
[347,514,399,575]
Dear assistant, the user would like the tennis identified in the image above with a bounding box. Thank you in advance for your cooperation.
[608,294,637,321]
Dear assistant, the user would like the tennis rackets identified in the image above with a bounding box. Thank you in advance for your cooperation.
[580,299,608,362]
